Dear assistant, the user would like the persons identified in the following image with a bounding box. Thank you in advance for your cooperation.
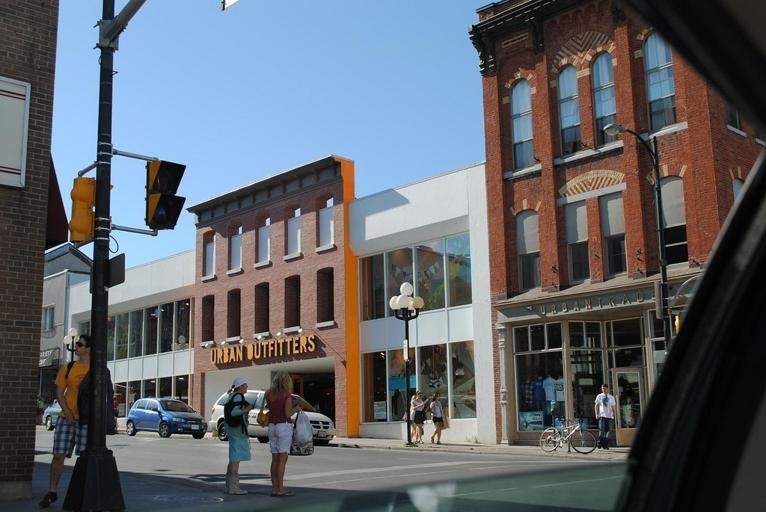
[226,377,253,495]
[403,391,444,444]
[594,383,617,450]
[264,369,302,496]
[522,371,565,429]
[39,333,95,507]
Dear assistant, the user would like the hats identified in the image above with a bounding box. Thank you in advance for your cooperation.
[232,376,248,389]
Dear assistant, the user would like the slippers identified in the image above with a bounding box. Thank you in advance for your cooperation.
[276,490,293,497]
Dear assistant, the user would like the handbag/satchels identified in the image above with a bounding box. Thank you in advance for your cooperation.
[443,416,448,428]
[290,409,313,456]
[256,396,269,426]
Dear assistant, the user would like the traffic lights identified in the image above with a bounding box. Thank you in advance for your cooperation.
[140,155,187,232]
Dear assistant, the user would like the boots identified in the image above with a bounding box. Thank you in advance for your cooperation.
[228,473,248,495]
[224,474,228,493]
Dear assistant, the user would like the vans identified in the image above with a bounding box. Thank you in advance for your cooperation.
[208,388,336,446]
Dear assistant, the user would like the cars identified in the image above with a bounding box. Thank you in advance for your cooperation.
[39,399,62,433]
[124,396,209,441]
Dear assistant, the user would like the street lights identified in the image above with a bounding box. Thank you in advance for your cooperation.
[61,326,82,360]
[387,281,426,448]
[600,122,672,356]
[169,335,189,351]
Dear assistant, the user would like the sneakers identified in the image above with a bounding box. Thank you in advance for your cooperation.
[430,437,433,443]
[38,491,57,508]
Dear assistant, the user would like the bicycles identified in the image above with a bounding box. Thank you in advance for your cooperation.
[537,413,599,456]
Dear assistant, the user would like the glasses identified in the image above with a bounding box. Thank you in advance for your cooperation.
[75,342,86,348]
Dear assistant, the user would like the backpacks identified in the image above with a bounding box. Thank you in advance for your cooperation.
[224,392,249,436]
